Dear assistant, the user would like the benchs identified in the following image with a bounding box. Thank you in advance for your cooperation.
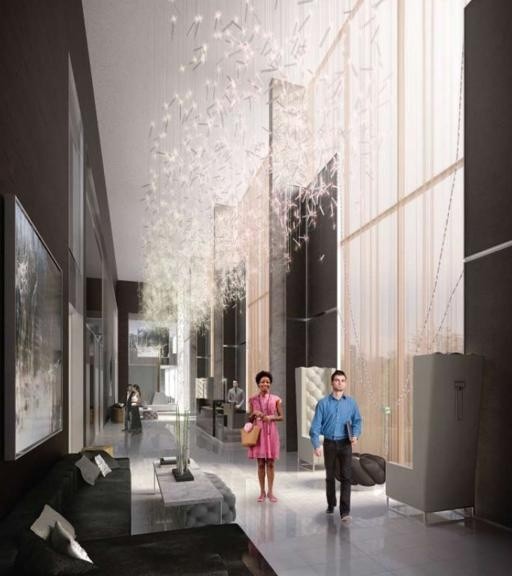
[175,472,237,530]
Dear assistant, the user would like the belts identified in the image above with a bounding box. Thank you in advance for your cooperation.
[325,505,353,522]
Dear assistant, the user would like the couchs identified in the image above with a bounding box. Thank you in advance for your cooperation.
[1,450,275,576]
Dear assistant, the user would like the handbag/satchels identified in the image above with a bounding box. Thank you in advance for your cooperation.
[241,422,261,448]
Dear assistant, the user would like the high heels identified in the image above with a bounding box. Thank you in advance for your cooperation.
[257,494,277,503]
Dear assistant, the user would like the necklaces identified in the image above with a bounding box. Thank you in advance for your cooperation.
[258,392,271,435]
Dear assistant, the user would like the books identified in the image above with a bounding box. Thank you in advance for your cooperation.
[347,420,353,442]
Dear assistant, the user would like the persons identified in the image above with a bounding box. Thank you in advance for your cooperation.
[246,370,285,504]
[128,384,144,434]
[228,379,246,413]
[121,383,133,430]
[309,369,362,521]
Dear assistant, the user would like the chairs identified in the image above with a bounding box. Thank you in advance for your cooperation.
[382,351,488,529]
[295,365,338,473]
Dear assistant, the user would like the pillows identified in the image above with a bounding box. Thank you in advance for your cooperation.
[74,451,123,487]
[29,503,94,564]
